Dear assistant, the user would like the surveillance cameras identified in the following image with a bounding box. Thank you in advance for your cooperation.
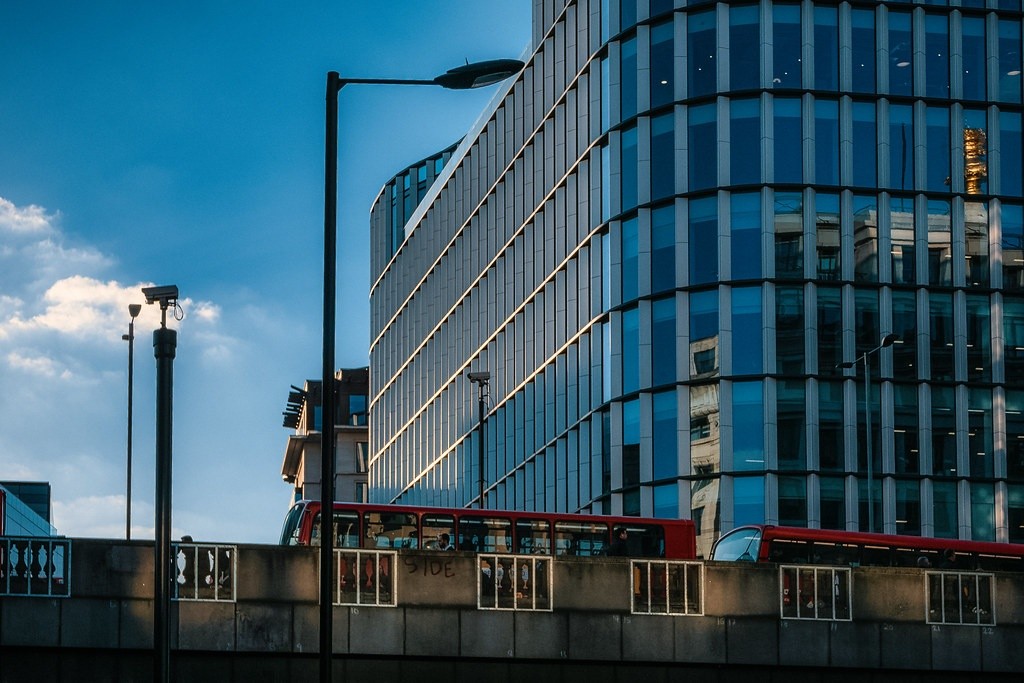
[467,372,490,381]
[142,285,178,301]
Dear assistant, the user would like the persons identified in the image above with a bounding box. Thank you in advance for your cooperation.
[916,555,930,568]
[438,533,455,551]
[937,548,968,612]
[607,528,631,556]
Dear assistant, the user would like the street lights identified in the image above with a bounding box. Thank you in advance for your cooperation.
[122,304,142,540]
[319,59,524,683]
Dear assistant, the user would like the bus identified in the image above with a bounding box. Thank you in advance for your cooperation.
[708,525,1024,625]
[284,504,697,604]
